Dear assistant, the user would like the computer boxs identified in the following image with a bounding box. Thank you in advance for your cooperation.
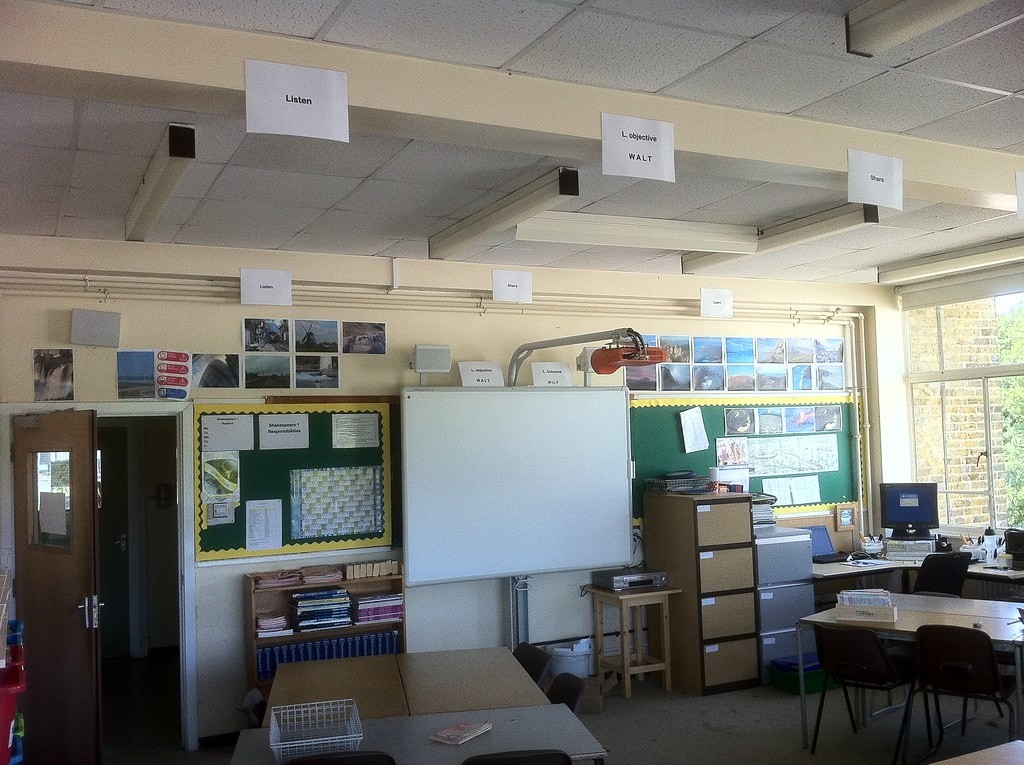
[887,539,936,553]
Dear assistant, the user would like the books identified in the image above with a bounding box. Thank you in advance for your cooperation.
[752,494,778,528]
[254,559,399,589]
[255,585,405,638]
[719,484,744,493]
[256,629,400,681]
[428,718,493,745]
[842,560,891,567]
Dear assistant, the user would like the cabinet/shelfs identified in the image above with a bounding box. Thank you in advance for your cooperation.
[243,558,407,694]
[752,526,818,685]
[644,491,759,694]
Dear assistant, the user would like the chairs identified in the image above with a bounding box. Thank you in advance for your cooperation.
[463,749,572,765]
[286,751,397,765]
[547,673,585,716]
[910,552,973,598]
[809,622,933,756]
[892,625,1014,765]
[513,642,553,693]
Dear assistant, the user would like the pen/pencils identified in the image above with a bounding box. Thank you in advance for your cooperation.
[970,538,974,544]
[978,534,985,546]
[997,538,1006,547]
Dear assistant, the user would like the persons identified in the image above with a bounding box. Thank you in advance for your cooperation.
[720,439,746,466]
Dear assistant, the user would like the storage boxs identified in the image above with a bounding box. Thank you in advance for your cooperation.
[998,557,1013,568]
[0,619,26,765]
[771,651,824,695]
[835,603,897,622]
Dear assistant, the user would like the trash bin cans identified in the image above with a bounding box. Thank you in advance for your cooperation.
[546,641,592,679]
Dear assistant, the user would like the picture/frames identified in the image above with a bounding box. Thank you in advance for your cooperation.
[836,504,857,531]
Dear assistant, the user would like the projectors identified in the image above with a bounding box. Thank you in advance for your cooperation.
[603,347,667,366]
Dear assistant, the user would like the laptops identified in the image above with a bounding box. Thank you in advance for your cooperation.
[801,524,860,563]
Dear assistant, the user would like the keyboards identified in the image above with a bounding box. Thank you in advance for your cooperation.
[885,552,930,560]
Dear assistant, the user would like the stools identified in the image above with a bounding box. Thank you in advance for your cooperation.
[581,585,683,698]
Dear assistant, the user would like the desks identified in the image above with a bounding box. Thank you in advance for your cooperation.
[795,590,1024,765]
[231,647,607,765]
[810,546,1024,607]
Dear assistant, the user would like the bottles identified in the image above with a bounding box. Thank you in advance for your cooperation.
[984,527,998,565]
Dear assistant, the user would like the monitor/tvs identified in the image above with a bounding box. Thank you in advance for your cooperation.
[879,483,939,540]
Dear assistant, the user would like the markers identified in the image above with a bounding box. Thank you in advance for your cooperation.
[961,533,967,545]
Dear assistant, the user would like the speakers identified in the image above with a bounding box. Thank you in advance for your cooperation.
[411,345,452,373]
[575,347,599,373]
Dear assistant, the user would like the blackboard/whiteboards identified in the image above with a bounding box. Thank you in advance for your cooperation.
[400,385,632,588]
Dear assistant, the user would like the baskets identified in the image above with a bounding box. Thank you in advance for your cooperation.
[268,698,364,765]
